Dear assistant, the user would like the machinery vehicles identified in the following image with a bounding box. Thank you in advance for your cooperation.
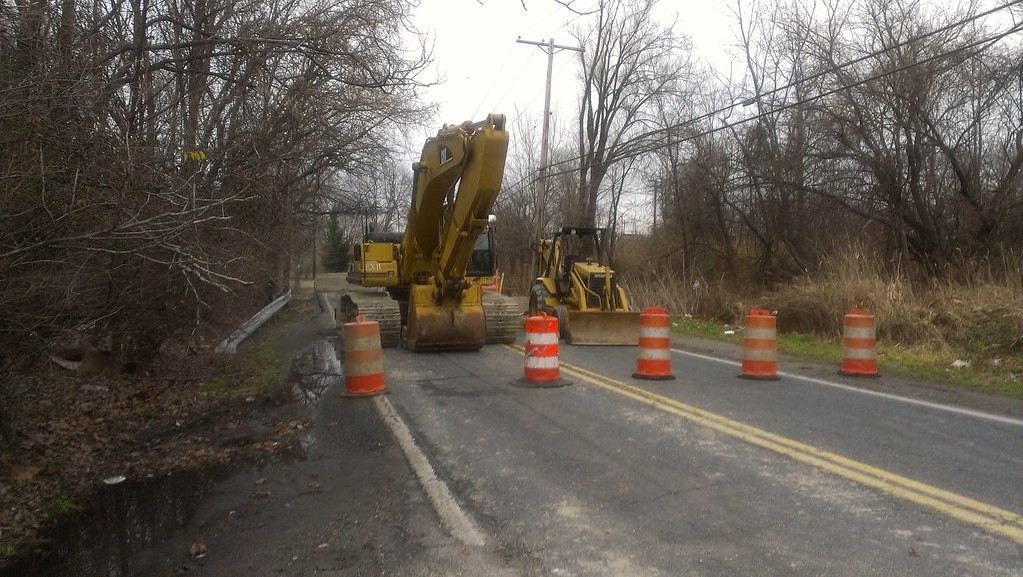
[528,226,642,346]
[340,113,520,352]
